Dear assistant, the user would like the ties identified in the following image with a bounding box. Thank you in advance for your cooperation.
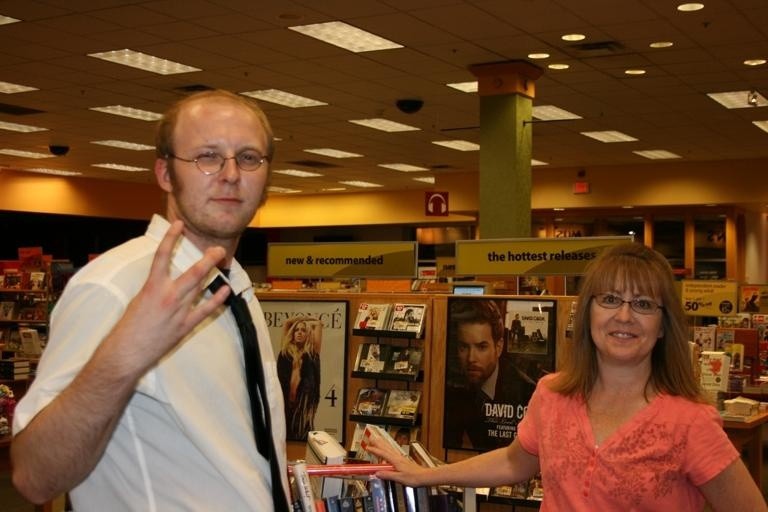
[208,274,293,512]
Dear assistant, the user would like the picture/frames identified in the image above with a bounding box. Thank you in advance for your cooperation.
[441,296,556,453]
[258,300,350,447]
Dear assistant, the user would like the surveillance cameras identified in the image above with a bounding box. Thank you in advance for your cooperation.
[49,145,70,157]
[396,99,423,114]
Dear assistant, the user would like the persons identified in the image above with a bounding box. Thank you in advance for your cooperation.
[8,87,295,512]
[443,297,538,450]
[278,315,326,465]
[512,311,526,351]
[363,240,767,511]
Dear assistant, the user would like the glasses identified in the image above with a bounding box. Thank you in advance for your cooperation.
[165,148,272,176]
[591,291,665,315]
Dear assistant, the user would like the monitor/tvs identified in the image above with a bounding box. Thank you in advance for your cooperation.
[304,429,349,500]
[452,281,496,295]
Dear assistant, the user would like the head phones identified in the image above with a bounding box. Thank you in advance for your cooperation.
[428,194,447,213]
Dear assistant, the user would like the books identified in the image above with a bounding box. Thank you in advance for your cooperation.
[348,299,428,422]
[0,247,72,512]
[285,422,544,512]
[250,278,455,296]
[685,268,767,422]
